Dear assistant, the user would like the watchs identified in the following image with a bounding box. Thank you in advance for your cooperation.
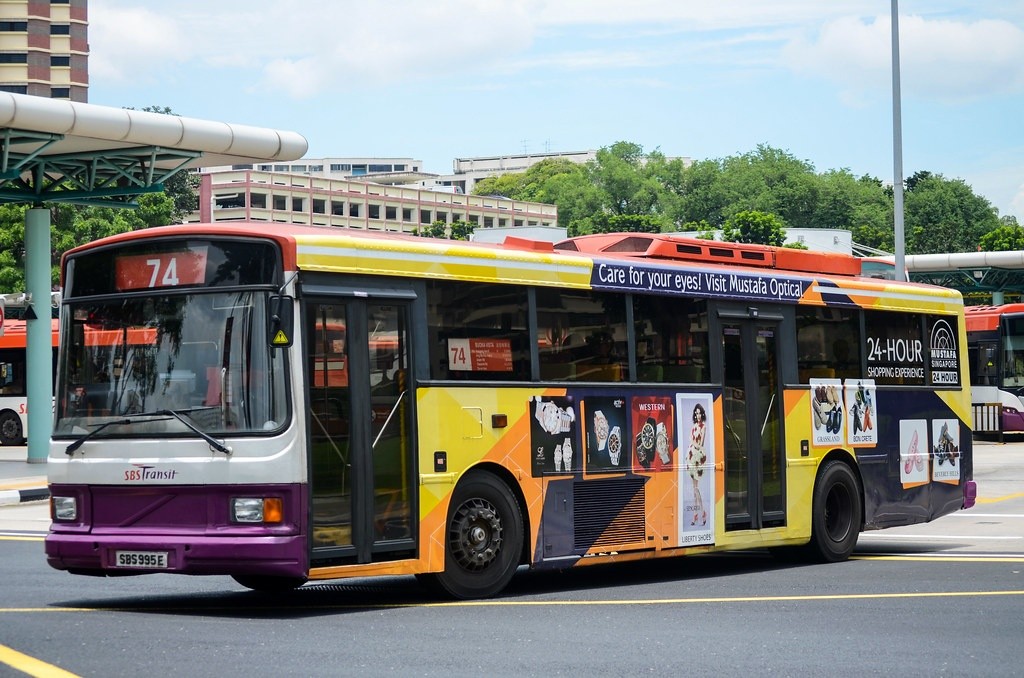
[536,401,572,434]
[593,410,609,451]
[607,425,622,466]
[636,417,671,470]
[563,438,573,472]
[554,444,562,472]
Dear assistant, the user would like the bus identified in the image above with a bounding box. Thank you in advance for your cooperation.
[965,303,1024,441]
[43,231,978,601]
[311,316,408,388]
[0,317,162,447]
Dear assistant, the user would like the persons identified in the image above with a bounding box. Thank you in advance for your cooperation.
[556,332,617,363]
[685,403,708,526]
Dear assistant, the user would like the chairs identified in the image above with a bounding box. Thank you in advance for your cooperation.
[208,364,905,492]
